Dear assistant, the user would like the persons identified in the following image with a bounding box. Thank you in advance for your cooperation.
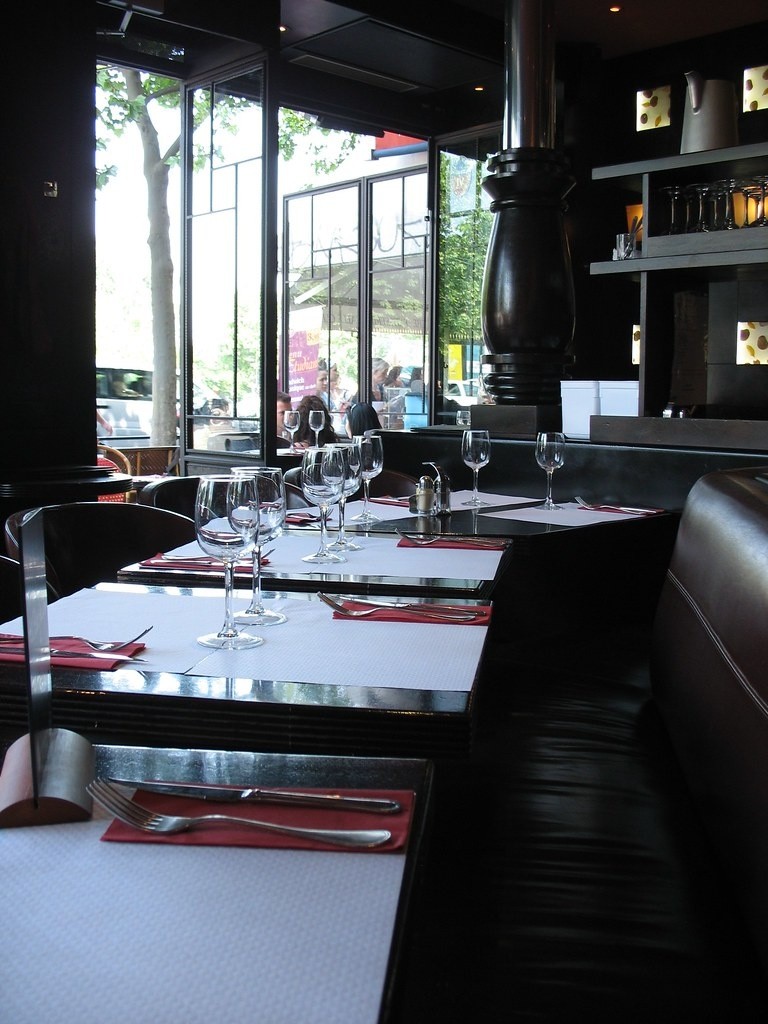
[193,358,422,450]
[112,372,152,398]
[97,410,112,438]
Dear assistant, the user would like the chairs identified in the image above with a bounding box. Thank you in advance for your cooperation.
[96,444,181,502]
[139,474,315,525]
[0,556,58,625]
[4,502,197,598]
[284,466,418,501]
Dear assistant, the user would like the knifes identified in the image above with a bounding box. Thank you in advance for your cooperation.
[150,559,266,568]
[0,646,148,663]
[107,774,401,813]
[337,594,486,615]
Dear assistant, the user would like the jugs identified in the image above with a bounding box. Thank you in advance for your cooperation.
[678,70,739,155]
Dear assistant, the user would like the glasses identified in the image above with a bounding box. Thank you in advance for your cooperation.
[350,402,367,414]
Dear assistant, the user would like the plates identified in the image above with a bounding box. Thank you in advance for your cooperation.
[410,424,470,435]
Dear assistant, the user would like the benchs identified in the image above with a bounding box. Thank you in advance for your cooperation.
[405,464,768,1023]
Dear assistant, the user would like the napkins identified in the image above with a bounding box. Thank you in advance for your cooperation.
[577,503,666,516]
[139,552,269,573]
[99,780,414,852]
[397,538,506,550]
[360,495,410,507]
[333,600,492,625]
[0,632,145,670]
[285,513,331,524]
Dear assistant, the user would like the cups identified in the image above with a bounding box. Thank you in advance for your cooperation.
[455,410,471,426]
[616,233,635,259]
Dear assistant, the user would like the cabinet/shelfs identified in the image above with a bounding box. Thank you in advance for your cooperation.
[590,134,768,452]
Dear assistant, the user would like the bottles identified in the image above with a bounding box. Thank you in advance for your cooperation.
[678,409,685,418]
[663,401,676,418]
[659,176,768,232]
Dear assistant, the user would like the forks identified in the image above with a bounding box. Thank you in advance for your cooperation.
[286,509,335,521]
[574,496,645,516]
[394,527,512,547]
[84,774,393,850]
[0,626,153,651]
[316,590,476,621]
[161,549,274,560]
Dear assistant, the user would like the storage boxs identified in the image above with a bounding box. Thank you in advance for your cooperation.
[560,379,639,439]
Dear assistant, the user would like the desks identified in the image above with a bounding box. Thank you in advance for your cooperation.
[0,470,133,510]
[0,742,433,1024]
[117,529,517,600]
[0,580,494,874]
[278,488,673,541]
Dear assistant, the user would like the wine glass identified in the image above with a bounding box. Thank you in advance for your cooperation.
[227,467,288,627]
[283,410,301,455]
[534,431,565,511]
[320,444,365,550]
[348,434,384,523]
[301,449,347,564]
[308,410,325,449]
[191,473,263,651]
[461,430,490,506]
